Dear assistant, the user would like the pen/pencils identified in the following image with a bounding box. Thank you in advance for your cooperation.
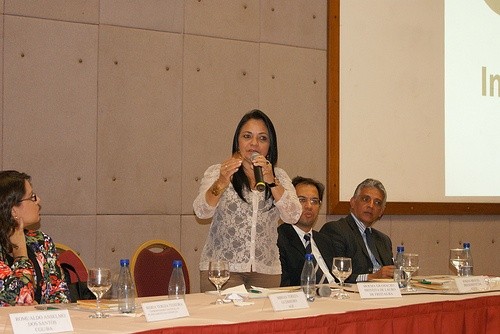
[419,280,443,285]
[34,306,60,309]
[247,289,263,293]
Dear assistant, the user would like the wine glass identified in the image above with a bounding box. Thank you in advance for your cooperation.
[208,261,230,305]
[400,254,419,292]
[87,268,112,318]
[332,258,352,299]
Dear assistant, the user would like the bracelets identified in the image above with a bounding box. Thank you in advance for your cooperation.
[210,179,228,196]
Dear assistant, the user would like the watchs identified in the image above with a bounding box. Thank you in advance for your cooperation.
[266,177,281,188]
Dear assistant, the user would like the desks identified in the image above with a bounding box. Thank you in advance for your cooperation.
[86,280,114,299]
[0,275,500,334]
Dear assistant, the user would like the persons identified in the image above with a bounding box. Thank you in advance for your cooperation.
[277,175,338,289]
[319,178,396,283]
[0,170,70,308]
[193,110,302,293]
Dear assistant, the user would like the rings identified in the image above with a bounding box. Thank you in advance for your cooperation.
[266,161,270,167]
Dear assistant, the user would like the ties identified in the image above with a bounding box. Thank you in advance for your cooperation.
[303,232,328,283]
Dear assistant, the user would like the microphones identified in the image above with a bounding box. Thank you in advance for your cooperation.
[251,152,265,192]
[315,285,331,297]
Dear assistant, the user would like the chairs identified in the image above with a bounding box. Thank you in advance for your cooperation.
[53,242,89,283]
[129,238,191,298]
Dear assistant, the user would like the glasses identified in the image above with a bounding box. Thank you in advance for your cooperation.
[298,198,322,206]
[16,193,37,203]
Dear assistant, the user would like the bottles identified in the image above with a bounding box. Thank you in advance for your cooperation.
[301,254,316,302]
[168,260,186,303]
[450,249,468,276]
[116,259,136,314]
[393,246,407,288]
[456,243,473,276]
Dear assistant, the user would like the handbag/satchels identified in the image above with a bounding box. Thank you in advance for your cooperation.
[60,263,95,303]
